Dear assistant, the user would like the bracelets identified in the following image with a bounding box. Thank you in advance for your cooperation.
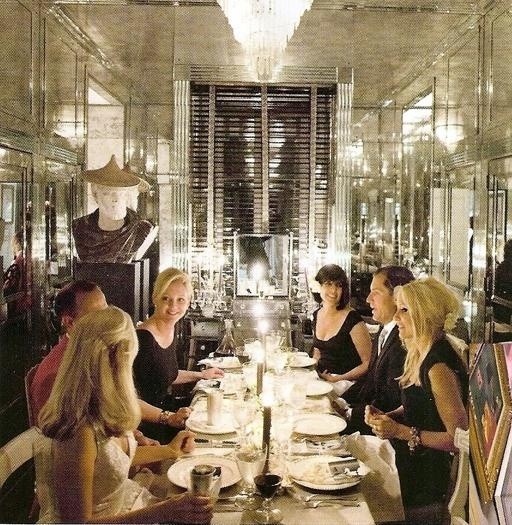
[160,409,171,426]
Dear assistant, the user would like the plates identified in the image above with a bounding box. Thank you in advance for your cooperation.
[168,454,240,492]
[291,456,368,491]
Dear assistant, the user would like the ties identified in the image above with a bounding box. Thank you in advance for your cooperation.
[377,327,389,356]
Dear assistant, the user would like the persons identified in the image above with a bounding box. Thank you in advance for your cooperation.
[37,306,216,524]
[31,280,196,450]
[131,267,225,445]
[307,264,372,399]
[332,266,416,434]
[2,232,24,293]
[71,153,161,263]
[490,238,512,342]
[364,277,470,508]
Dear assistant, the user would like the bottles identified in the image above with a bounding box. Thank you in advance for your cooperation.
[211,319,241,357]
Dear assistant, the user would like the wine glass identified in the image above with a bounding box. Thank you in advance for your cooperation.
[251,459,285,524]
[233,439,268,513]
[184,333,347,437]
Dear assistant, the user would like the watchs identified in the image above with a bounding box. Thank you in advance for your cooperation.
[407,426,419,454]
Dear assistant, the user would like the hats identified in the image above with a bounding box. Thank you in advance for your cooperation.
[78,153,141,190]
[121,161,151,195]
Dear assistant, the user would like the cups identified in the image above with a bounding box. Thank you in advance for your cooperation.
[189,466,215,499]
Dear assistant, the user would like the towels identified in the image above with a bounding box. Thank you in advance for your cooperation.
[320,371,357,395]
[341,431,406,524]
[130,466,169,511]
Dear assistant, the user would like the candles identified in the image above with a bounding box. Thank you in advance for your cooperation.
[258,385,273,476]
[253,350,265,397]
[258,321,270,371]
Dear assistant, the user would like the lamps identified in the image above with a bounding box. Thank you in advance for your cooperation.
[214,0,313,84]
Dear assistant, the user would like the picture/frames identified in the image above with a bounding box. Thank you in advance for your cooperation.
[470,342,512,511]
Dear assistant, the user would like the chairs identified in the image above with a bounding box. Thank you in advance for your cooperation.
[0,426,46,524]
[25,364,43,427]
[444,333,469,373]
[380,442,470,525]
[362,313,384,367]
